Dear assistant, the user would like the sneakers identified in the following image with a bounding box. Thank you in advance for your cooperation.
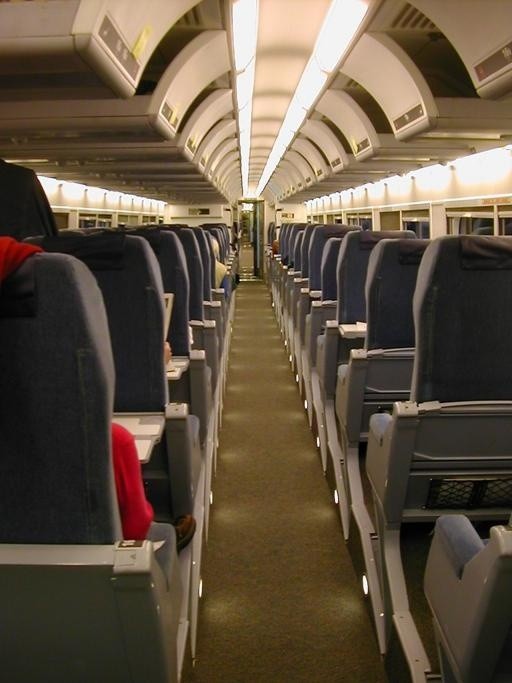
[175,514,196,552]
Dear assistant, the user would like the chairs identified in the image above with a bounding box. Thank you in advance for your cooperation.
[1,225,243,680]
[263,221,509,682]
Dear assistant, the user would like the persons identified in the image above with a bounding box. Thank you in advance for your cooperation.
[211,239,229,296]
[164,342,173,364]
[111,422,197,551]
